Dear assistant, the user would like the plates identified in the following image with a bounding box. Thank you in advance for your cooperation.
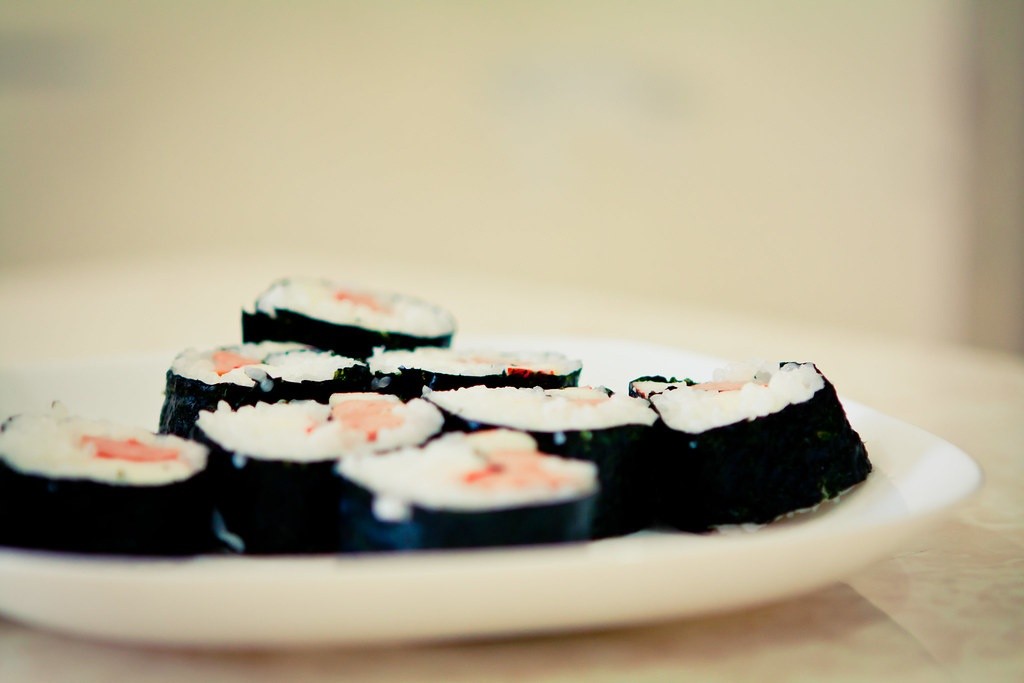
[0,339,980,648]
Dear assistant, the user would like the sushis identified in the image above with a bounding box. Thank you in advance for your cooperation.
[0,274,875,557]
